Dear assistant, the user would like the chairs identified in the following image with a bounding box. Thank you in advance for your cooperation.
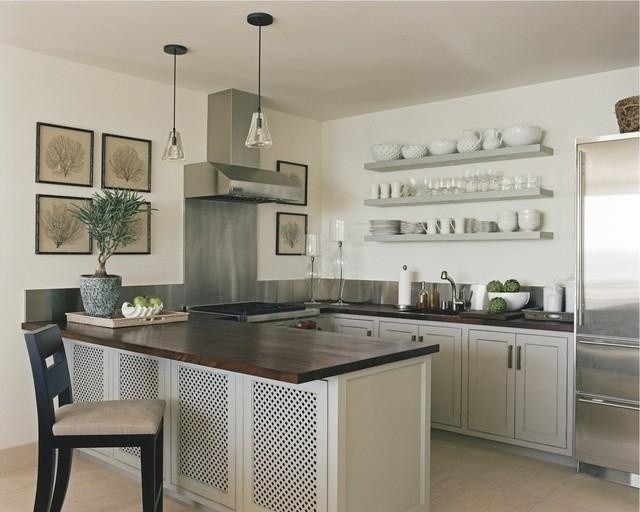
[23,324,168,512]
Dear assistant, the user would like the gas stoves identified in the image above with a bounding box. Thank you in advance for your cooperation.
[187,302,322,332]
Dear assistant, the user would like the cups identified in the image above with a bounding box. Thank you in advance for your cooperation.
[421,217,465,234]
[371,183,406,199]
[503,175,541,191]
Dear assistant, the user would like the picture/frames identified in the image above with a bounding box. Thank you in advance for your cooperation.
[275,211,308,256]
[113,201,152,255]
[101,132,153,193]
[276,160,308,207]
[35,193,94,256]
[33,121,96,189]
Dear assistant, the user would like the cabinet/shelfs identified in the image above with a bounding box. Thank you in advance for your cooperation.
[468,329,568,449]
[379,321,462,428]
[333,317,374,337]
[364,142,553,243]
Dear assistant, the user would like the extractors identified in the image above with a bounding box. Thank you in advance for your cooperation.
[184,88,302,205]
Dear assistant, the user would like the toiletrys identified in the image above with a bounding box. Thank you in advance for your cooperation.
[418,282,428,308]
[429,282,439,308]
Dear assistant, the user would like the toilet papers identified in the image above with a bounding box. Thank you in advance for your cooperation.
[398,265,410,308]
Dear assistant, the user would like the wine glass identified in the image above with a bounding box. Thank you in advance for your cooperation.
[424,168,502,197]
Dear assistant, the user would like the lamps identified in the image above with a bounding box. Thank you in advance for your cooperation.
[161,43,188,162]
[245,12,273,147]
[304,217,322,305]
[329,219,350,306]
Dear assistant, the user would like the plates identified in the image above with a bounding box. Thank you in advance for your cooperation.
[369,220,425,236]
[472,221,497,233]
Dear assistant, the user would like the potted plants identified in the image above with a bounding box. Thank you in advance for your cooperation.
[67,186,160,316]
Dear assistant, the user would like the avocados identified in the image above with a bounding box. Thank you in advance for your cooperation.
[488,297,506,313]
[504,279,521,291]
[486,279,503,292]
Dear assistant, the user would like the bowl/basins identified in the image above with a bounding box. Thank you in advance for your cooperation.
[488,291,530,312]
[370,144,403,162]
[497,209,541,231]
[502,125,542,146]
[402,145,428,159]
[428,140,458,156]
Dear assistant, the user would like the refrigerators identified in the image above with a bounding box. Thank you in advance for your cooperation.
[574,130,639,492]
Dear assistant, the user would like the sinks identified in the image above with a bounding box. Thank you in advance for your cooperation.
[399,308,460,316]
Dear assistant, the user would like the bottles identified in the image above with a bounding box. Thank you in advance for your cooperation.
[428,282,440,311]
[480,127,503,150]
[417,281,429,309]
[456,128,482,153]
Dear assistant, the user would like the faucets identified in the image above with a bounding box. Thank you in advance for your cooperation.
[441,271,463,311]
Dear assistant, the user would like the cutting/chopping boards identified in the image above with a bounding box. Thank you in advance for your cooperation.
[459,310,522,321]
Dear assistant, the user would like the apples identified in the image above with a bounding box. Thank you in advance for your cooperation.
[133,296,148,307]
[150,297,163,306]
